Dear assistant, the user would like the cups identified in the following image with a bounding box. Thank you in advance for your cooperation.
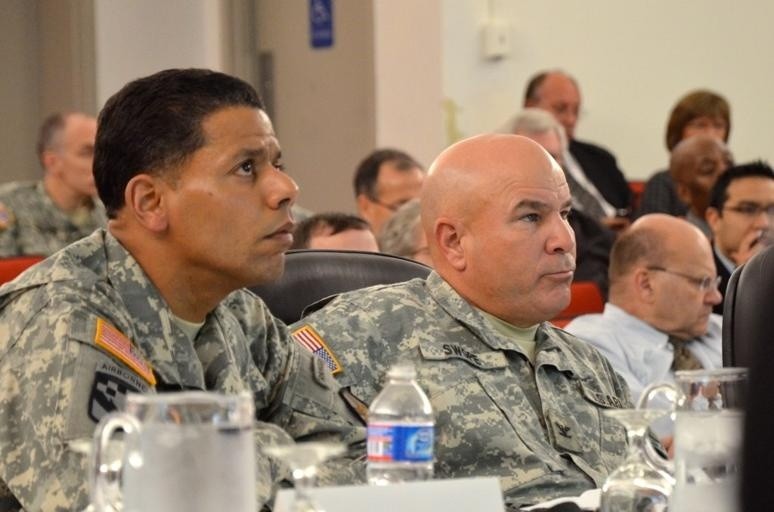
[88,389,259,511]
[634,366,748,482]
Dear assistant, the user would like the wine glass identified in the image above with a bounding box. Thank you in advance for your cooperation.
[600,406,676,512]
[262,438,350,510]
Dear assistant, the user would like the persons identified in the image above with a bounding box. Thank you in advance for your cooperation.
[282,134,671,512]
[377,202,435,272]
[286,208,383,258]
[0,67,370,512]
[351,147,428,235]
[561,211,726,412]
[667,135,737,241]
[634,90,730,219]
[701,159,773,315]
[524,69,636,234]
[502,114,620,308]
[0,109,114,261]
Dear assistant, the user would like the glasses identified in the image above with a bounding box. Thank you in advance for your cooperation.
[646,265,721,292]
[723,200,774,214]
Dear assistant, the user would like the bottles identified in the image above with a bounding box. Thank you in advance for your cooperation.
[361,361,437,484]
